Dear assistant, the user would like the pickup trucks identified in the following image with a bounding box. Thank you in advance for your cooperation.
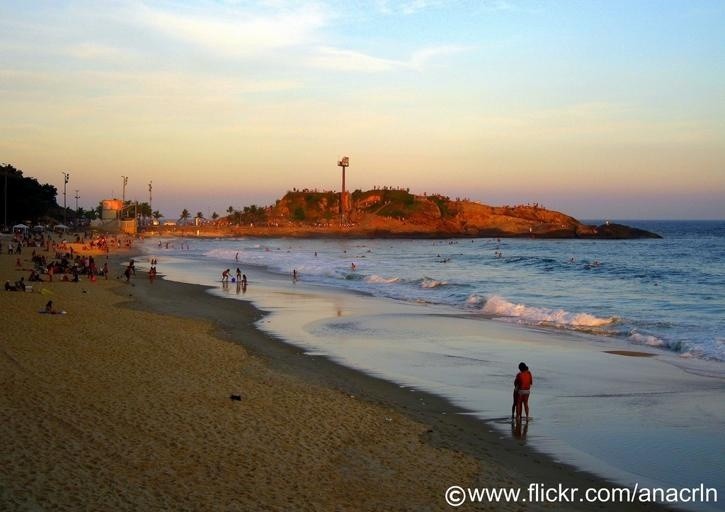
[162,221,175,226]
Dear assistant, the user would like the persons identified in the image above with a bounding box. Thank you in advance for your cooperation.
[292,269,299,282]
[3,226,176,295]
[44,300,63,315]
[235,252,239,262]
[510,366,533,419]
[351,262,355,269]
[241,274,247,284]
[430,236,505,264]
[234,267,242,281]
[513,362,533,421]
[221,268,232,282]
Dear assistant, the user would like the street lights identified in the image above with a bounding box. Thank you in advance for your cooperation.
[119,176,128,201]
[61,172,70,226]
[1,162,7,231]
[147,179,152,225]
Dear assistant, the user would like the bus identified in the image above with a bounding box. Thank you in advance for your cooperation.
[8,224,67,243]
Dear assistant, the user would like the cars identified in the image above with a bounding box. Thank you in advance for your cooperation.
[67,217,89,228]
[149,219,160,225]
